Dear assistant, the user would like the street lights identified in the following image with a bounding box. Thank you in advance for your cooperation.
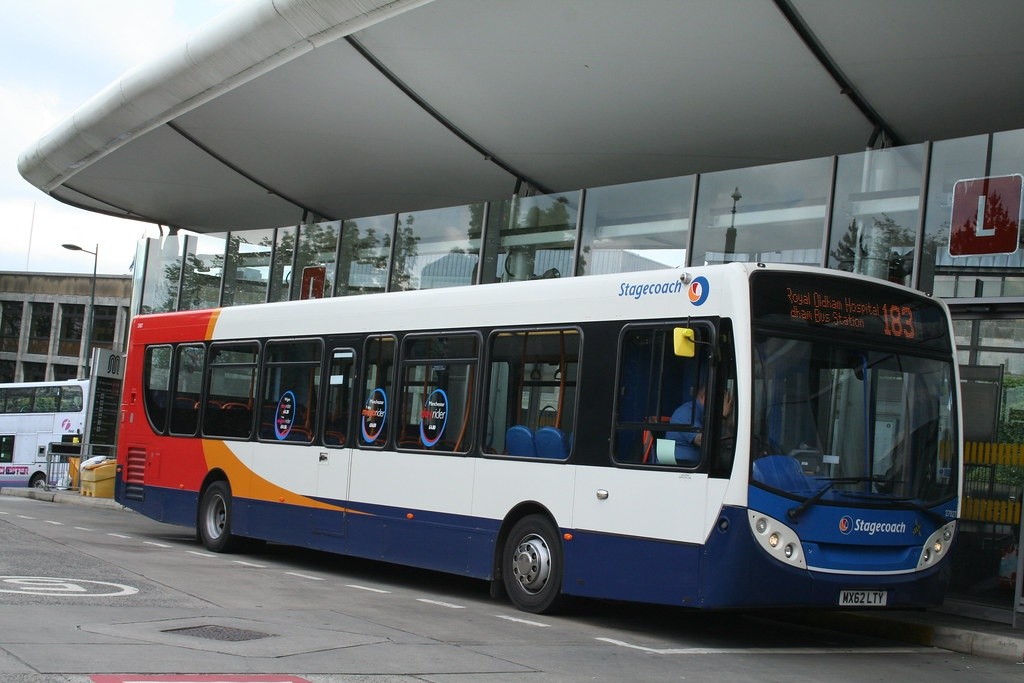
[61,242,99,377]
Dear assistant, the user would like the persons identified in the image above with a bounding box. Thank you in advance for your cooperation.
[665,383,733,466]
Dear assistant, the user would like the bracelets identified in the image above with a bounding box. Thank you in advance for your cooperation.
[722,415,727,420]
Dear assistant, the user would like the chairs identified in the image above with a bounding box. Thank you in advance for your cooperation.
[174,396,499,454]
[533,426,567,459]
[644,415,701,467]
[505,425,536,458]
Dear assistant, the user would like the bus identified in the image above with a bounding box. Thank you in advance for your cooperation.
[114,258,965,616]
[0,379,90,490]
